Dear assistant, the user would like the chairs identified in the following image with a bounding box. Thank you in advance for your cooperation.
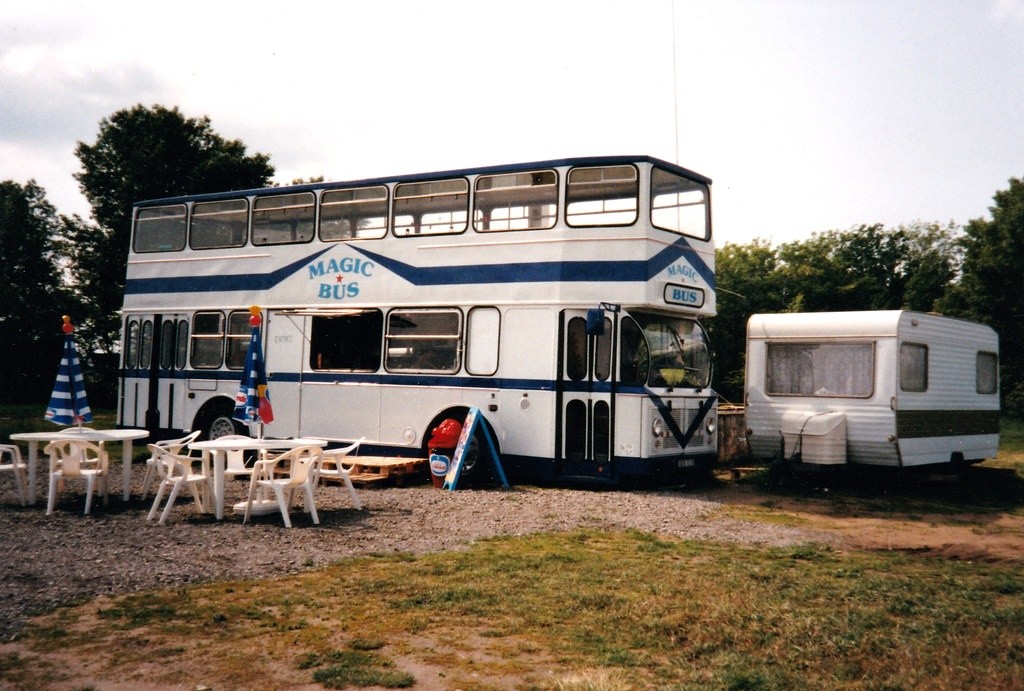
[48,427,106,498]
[285,435,365,512]
[146,443,215,526]
[43,438,110,514]
[242,444,325,528]
[139,429,202,500]
[211,435,268,513]
[0,444,29,507]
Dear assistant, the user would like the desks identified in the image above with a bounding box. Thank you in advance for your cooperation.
[10,429,151,505]
[187,437,329,520]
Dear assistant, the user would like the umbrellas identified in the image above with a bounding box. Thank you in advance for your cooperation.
[230,306,274,441]
[44,316,93,434]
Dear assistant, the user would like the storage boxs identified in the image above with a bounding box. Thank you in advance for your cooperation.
[781,410,848,465]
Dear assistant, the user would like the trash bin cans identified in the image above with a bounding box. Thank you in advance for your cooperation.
[427,439,457,489]
[779,408,846,466]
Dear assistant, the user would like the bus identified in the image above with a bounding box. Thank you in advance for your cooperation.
[115,155,719,489]
